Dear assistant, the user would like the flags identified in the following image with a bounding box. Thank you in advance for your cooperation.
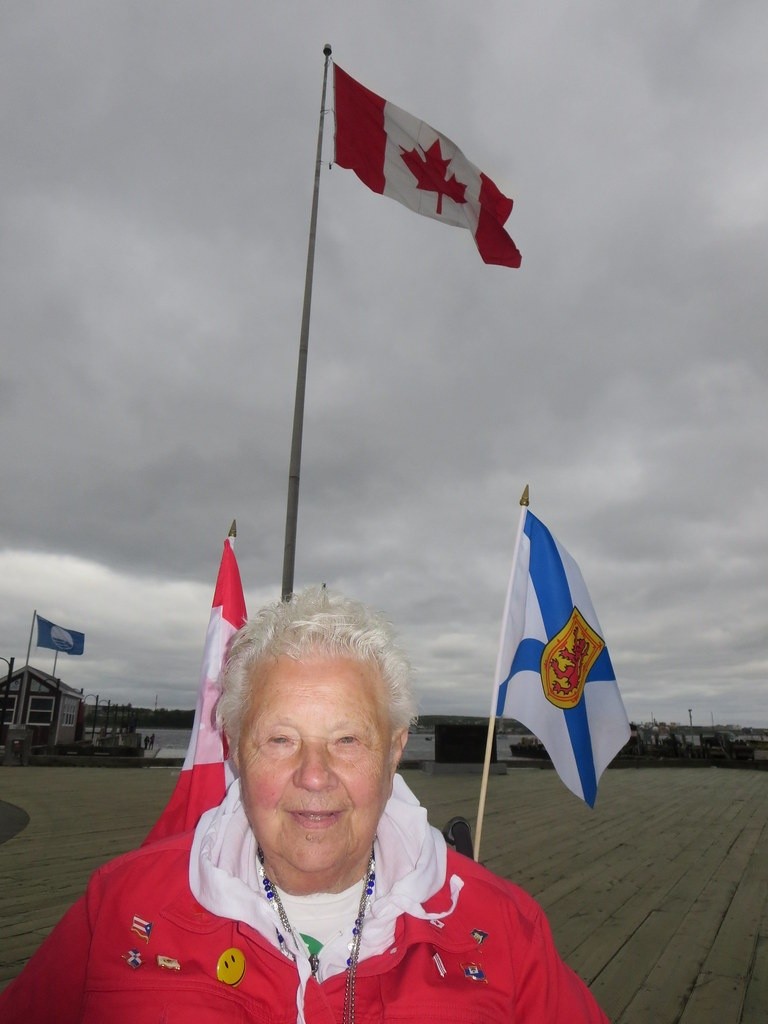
[331,62,523,270]
[138,541,249,849]
[37,614,84,655]
[495,510,632,809]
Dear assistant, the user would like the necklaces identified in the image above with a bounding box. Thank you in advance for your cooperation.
[258,841,375,1024]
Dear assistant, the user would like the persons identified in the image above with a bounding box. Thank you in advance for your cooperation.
[144,736,149,749]
[0,583,610,1024]
[149,733,155,750]
